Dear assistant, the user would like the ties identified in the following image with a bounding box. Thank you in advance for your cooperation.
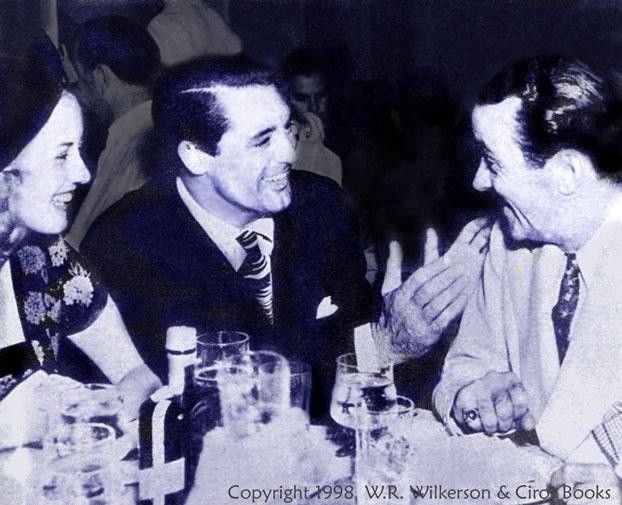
[552,253,580,366]
[235,229,275,324]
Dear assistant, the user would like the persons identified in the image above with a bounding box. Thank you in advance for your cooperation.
[1,1,621,504]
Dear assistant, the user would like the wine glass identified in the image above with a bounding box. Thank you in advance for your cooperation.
[1,326,415,504]
